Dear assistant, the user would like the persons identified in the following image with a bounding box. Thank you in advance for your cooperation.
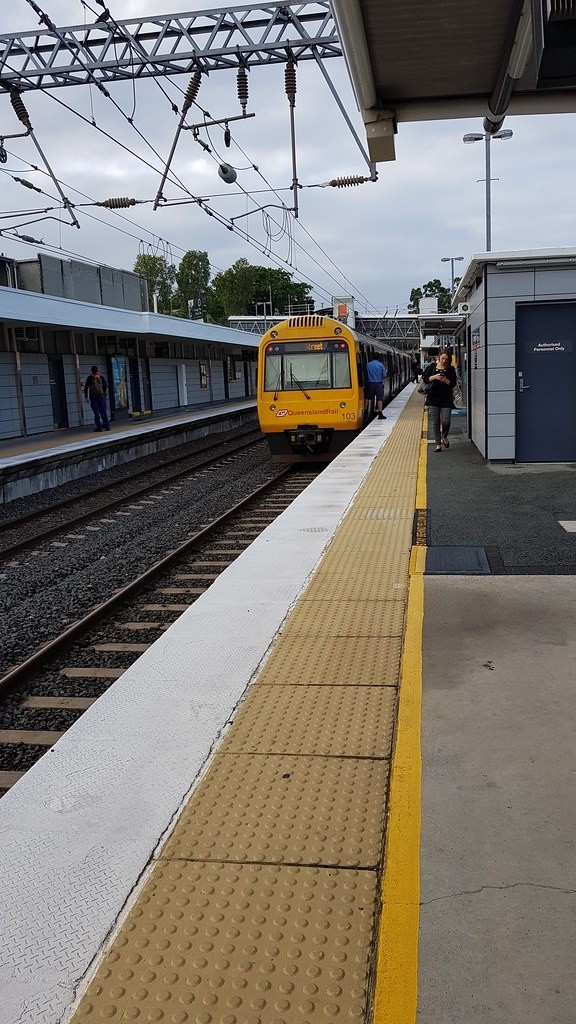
[83,365,112,432]
[409,356,464,390]
[366,353,387,420]
[421,349,458,453]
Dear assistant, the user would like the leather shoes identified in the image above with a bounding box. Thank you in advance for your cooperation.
[434,447,441,452]
[443,439,449,448]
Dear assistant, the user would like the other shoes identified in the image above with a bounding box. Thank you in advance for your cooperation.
[94,428,102,432]
[378,416,386,419]
[106,426,112,431]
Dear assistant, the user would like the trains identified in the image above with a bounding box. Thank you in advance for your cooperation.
[255,315,419,456]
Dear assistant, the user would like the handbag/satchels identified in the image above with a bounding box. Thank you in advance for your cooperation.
[418,380,433,395]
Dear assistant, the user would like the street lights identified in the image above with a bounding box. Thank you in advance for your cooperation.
[462,128,514,253]
[441,256,465,298]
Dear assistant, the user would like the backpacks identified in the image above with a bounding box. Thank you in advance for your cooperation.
[90,374,103,396]
